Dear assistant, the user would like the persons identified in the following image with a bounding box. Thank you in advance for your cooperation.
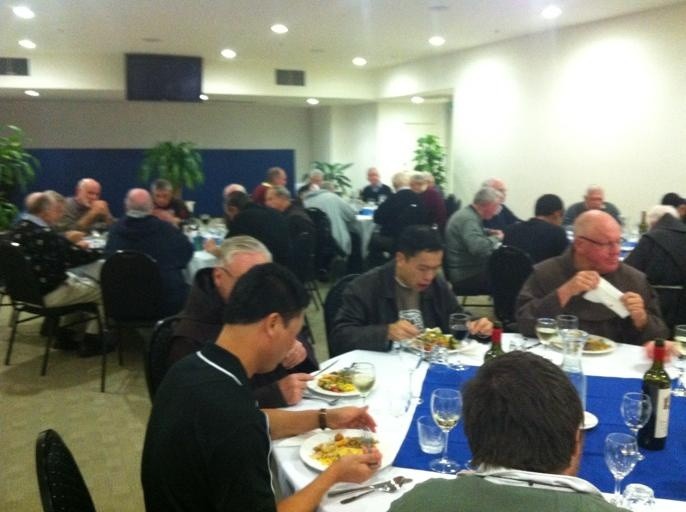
[8,167,193,359]
[140,167,686,512]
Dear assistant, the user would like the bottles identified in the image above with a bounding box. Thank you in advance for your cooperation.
[484,319,505,365]
[639,338,670,450]
[561,329,586,415]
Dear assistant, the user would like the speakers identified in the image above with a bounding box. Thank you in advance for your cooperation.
[0,57,31,76]
[276,69,304,86]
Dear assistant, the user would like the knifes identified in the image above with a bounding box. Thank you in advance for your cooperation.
[327,479,412,498]
[312,360,338,378]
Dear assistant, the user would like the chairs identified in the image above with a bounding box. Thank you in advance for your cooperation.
[34,426,99,511]
[98,247,183,393]
[491,246,537,330]
[1,238,107,376]
[303,246,324,310]
[147,311,188,410]
[651,282,683,343]
[440,250,504,329]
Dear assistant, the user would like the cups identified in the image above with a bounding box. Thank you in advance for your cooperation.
[559,313,579,348]
[509,334,528,353]
[398,308,425,335]
[417,414,444,455]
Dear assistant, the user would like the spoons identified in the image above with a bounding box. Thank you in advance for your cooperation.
[340,476,404,504]
[303,395,343,406]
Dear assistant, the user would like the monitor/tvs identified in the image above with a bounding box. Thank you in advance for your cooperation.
[123,52,204,103]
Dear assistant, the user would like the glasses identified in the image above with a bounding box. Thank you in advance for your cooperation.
[580,235,627,251]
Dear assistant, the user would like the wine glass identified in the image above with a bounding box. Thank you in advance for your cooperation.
[670,325,686,398]
[448,314,471,371]
[431,388,463,473]
[604,393,654,511]
[351,361,376,407]
[398,339,424,406]
[535,317,558,358]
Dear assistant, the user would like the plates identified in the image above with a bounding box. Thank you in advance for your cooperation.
[557,333,617,355]
[580,411,599,432]
[411,334,479,354]
[307,368,380,397]
[299,428,396,473]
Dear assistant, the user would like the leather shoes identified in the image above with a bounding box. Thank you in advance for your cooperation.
[39,322,113,357]
